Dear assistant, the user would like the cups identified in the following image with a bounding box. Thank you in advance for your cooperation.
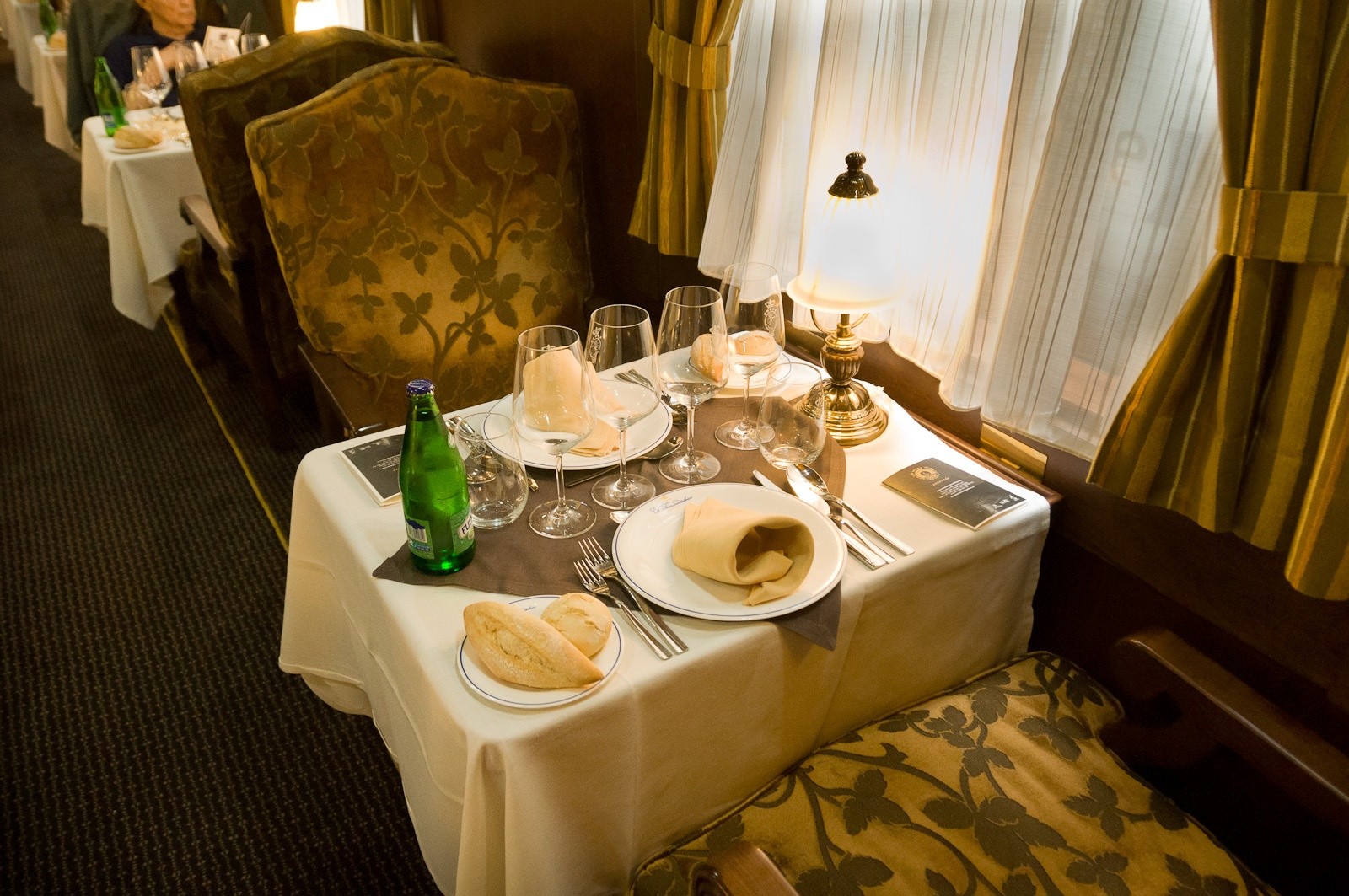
[454,412,529,530]
[174,33,270,85]
[57,6,70,33]
[756,361,826,471]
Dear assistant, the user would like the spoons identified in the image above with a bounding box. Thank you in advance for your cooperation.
[609,511,631,524]
[564,435,684,488]
[786,462,916,564]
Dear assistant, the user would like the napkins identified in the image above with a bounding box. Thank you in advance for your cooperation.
[673,494,816,610]
[526,350,628,458]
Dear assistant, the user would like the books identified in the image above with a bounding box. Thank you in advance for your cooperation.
[882,457,1028,531]
[202,13,252,62]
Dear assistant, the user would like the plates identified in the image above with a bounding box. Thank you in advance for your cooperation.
[457,595,626,710]
[612,483,848,622]
[109,104,184,154]
[481,343,792,471]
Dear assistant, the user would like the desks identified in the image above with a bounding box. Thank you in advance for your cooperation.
[260,330,1052,896]
[0,0,81,162]
[82,106,209,330]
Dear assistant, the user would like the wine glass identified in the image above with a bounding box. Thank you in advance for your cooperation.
[512,262,784,540]
[131,45,184,141]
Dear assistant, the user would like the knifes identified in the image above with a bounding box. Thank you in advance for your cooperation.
[752,470,887,571]
[450,414,538,492]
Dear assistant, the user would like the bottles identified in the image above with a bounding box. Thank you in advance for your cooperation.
[38,0,57,43]
[398,379,476,577]
[94,57,129,138]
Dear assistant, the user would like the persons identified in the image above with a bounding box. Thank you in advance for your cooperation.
[48,0,72,25]
[101,0,254,112]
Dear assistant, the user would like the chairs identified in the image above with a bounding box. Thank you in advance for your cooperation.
[245,56,599,444]
[63,0,285,145]
[174,25,461,452]
[630,630,1349,896]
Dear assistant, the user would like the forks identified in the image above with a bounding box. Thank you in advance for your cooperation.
[614,369,699,426]
[572,536,689,660]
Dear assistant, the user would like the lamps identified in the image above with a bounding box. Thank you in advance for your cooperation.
[786,151,898,446]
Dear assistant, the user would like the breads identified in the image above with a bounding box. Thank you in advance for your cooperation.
[463,591,612,688]
[113,125,162,149]
[690,331,775,381]
